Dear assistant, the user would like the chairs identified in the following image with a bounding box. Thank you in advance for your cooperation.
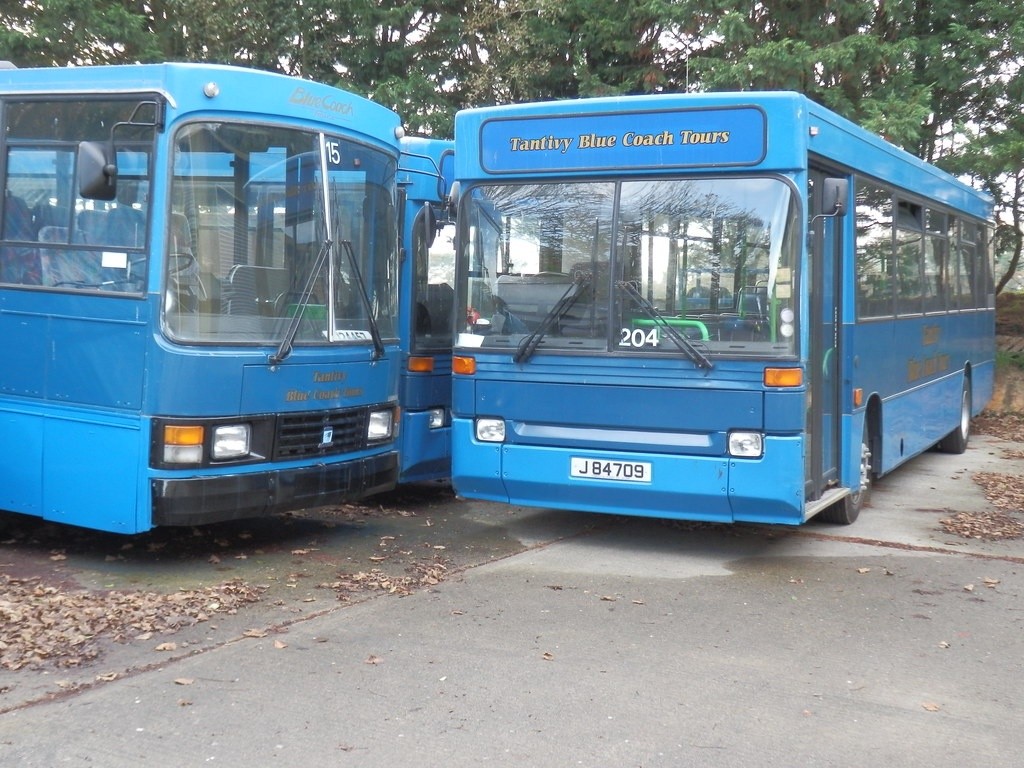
[570,261,626,302]
[717,287,733,307]
[0,195,200,314]
[686,287,709,309]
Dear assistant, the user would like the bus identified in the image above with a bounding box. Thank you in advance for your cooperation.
[436,93,996,525]
[4,137,502,489]
[0,60,446,535]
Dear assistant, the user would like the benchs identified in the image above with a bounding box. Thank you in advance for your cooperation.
[717,286,767,341]
